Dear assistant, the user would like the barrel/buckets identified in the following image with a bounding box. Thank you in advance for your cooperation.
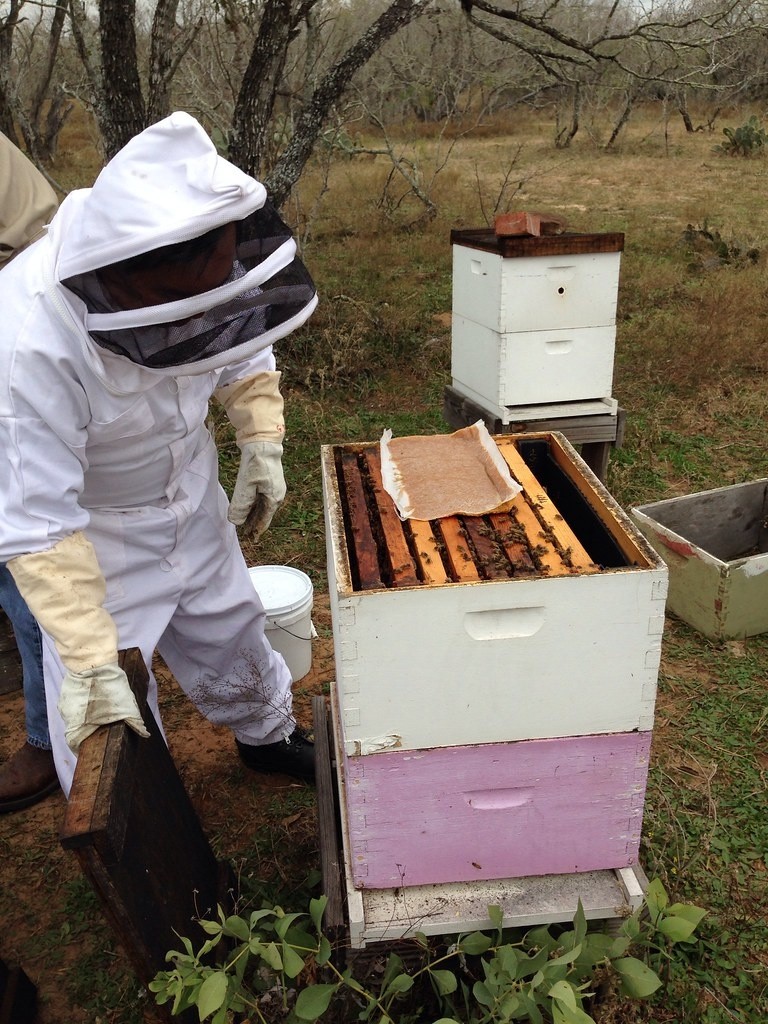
[246,566,320,683]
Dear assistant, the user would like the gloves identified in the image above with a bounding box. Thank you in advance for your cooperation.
[213,371,287,545]
[5,531,152,756]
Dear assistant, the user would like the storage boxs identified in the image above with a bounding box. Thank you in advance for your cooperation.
[631,475,768,644]
[327,682,653,894]
[321,428,670,758]
[450,311,617,408]
[449,225,625,334]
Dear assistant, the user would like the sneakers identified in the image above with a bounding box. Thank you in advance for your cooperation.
[234,724,316,784]
[0,740,60,813]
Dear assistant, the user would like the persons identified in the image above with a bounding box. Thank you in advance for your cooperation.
[0,132,68,816]
[0,112,319,850]
